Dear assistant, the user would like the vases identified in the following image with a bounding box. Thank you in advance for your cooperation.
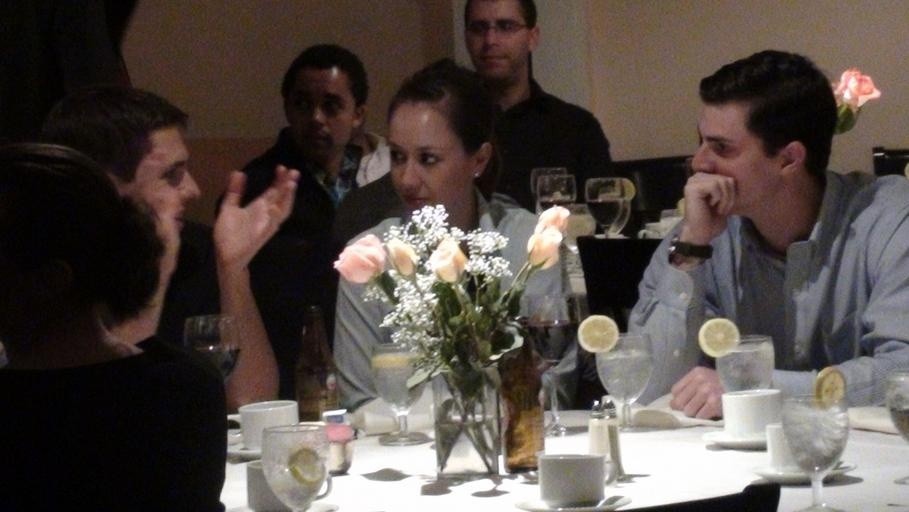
[429,366,506,490]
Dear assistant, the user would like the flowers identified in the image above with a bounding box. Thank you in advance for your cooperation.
[332,191,575,466]
[829,67,881,134]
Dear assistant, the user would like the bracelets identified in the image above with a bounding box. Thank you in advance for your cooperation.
[667,235,715,266]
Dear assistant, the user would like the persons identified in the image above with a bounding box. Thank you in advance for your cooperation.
[33,83,304,414]
[3,142,229,510]
[332,53,578,411]
[458,0,617,334]
[622,46,908,423]
[212,42,405,399]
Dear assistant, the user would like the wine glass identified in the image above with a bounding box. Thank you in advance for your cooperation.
[526,161,635,240]
[593,332,663,433]
[524,290,581,437]
[368,341,436,446]
[885,368,909,489]
[779,397,850,510]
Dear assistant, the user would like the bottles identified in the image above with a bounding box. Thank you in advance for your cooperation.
[323,408,348,426]
[292,280,340,423]
[604,402,633,482]
[586,402,614,484]
[500,355,548,473]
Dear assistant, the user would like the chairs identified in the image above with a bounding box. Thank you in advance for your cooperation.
[577,234,663,333]
[873,144,907,177]
[612,154,701,226]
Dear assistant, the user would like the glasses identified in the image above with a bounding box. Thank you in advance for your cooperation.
[472,20,524,32]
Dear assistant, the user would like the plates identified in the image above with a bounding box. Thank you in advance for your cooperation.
[226,442,265,461]
[710,436,769,453]
[514,494,631,512]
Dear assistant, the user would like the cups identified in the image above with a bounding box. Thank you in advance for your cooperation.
[721,388,787,446]
[260,423,328,510]
[325,421,358,476]
[535,453,608,508]
[180,311,244,379]
[715,333,778,390]
[245,463,332,511]
[765,422,789,459]
[226,397,298,449]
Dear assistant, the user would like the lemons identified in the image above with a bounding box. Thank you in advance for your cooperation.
[577,315,619,353]
[288,448,322,485]
[698,319,740,357]
[815,367,846,405]
[614,178,636,201]
